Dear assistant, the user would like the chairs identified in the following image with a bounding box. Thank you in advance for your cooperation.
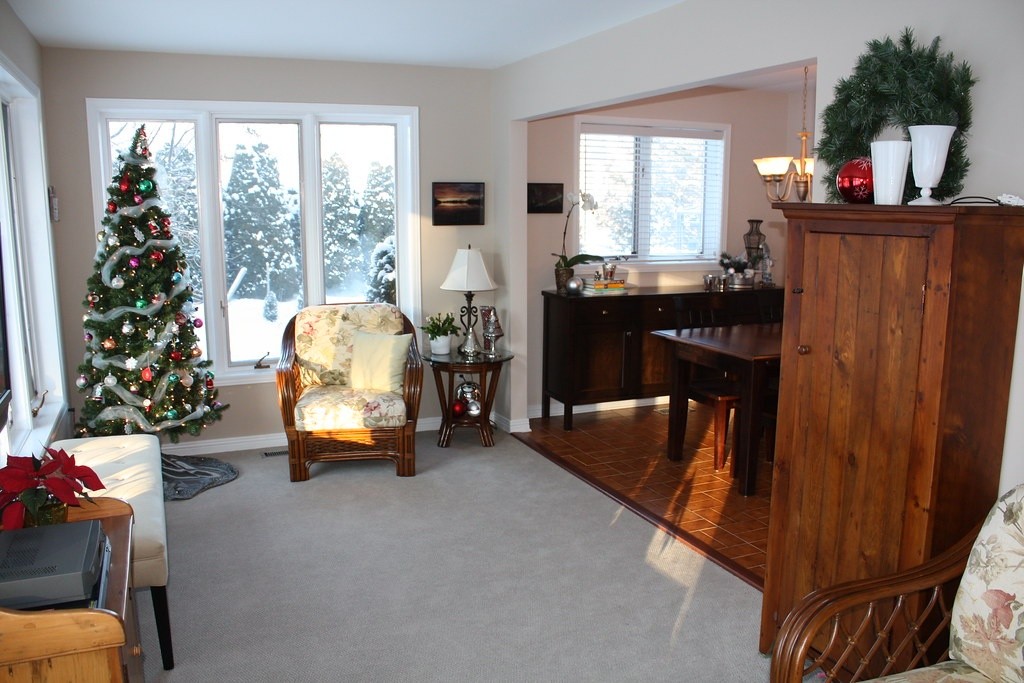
[769,483,1024,682]
[274,302,424,482]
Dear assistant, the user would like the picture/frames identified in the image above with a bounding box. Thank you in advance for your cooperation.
[432,182,485,226]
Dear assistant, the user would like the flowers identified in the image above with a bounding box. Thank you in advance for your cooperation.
[0,447,105,531]
[550,189,604,267]
[416,312,461,339]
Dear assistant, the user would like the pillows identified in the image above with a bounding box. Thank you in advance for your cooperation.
[350,330,413,396]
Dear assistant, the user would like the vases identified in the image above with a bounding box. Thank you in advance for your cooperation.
[528,183,564,213]
[555,268,574,293]
[908,124,957,207]
[19,483,67,528]
[743,218,766,257]
[869,140,911,205]
[430,334,451,354]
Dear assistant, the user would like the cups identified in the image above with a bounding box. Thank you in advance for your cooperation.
[602,263,616,280]
[870,140,912,206]
[717,275,730,290]
[703,274,716,290]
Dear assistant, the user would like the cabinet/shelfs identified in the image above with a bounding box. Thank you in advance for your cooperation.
[0,498,146,683]
[756,200,1024,646]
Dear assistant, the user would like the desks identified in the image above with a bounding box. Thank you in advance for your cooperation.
[647,326,780,497]
[44,433,175,671]
[540,281,785,432]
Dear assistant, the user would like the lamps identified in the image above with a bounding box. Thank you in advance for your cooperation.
[438,243,498,356]
[752,67,814,202]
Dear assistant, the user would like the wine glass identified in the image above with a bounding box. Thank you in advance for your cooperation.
[907,124,957,206]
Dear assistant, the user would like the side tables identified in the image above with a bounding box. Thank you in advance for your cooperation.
[422,347,514,448]
